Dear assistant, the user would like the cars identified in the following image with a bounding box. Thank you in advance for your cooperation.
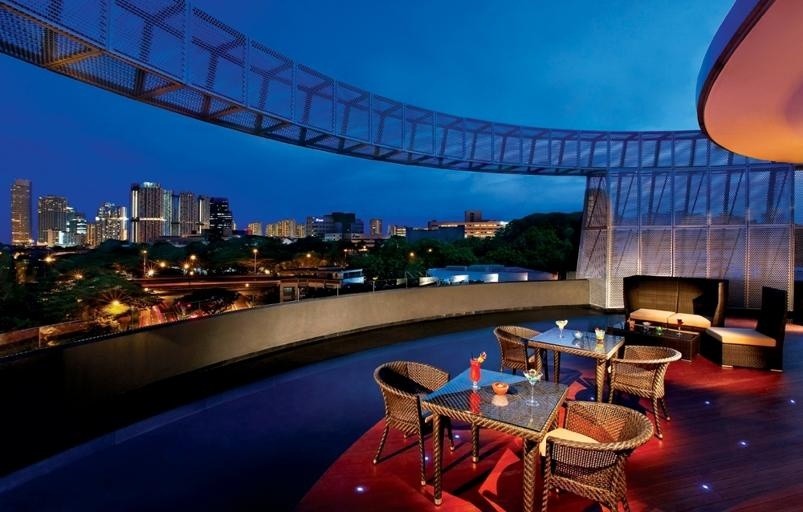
[177,296,223,313]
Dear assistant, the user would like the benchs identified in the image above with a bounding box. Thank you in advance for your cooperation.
[704,286,787,372]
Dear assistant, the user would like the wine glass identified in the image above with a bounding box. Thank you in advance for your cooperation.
[555,320,568,338]
[469,358,480,387]
[469,387,480,414]
[525,404,540,430]
[522,370,543,404]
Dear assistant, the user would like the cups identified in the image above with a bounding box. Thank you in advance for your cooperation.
[594,330,605,341]
[594,341,605,353]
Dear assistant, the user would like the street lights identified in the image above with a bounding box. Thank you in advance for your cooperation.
[253,248,258,272]
[143,250,147,272]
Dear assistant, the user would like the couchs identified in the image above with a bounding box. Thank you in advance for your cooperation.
[622,275,729,342]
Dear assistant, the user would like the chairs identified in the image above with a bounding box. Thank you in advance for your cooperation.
[607,345,683,438]
[542,399,654,512]
[492,325,549,380]
[372,360,455,485]
